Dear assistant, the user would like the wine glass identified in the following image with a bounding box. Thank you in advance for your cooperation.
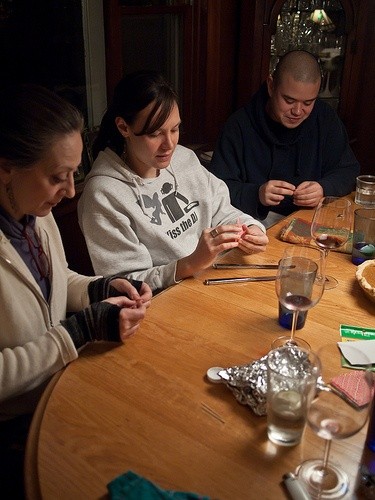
[267,245,325,355]
[296,343,372,498]
[311,196,354,290]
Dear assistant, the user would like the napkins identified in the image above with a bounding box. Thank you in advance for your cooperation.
[106,472,210,500]
[280,218,353,254]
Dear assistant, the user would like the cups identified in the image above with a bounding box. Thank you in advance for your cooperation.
[354,175,375,208]
[267,346,322,446]
[353,209,375,266]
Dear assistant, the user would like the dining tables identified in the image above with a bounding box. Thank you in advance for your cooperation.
[22,191,375,500]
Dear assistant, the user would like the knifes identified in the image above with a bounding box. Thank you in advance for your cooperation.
[213,263,296,269]
[206,276,280,284]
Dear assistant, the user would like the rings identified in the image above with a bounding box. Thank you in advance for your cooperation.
[210,229,219,237]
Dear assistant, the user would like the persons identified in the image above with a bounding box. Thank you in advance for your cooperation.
[0,83,152,422]
[207,50,361,230]
[76,74,270,292]
